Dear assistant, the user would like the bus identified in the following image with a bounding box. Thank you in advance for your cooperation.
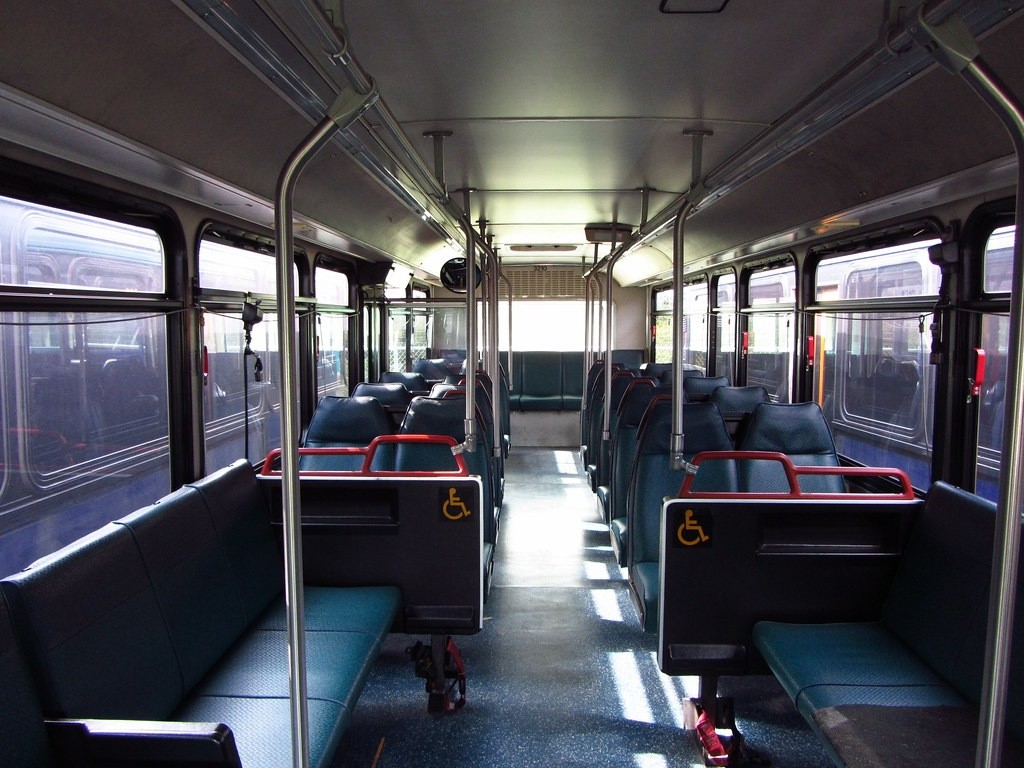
[1,195,433,580]
[677,226,1024,518]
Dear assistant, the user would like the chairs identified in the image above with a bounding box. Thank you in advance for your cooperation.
[299,359,511,635]
[582,360,850,633]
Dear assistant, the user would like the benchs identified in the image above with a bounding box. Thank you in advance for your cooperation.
[0,458,401,768]
[751,480,1024,768]
